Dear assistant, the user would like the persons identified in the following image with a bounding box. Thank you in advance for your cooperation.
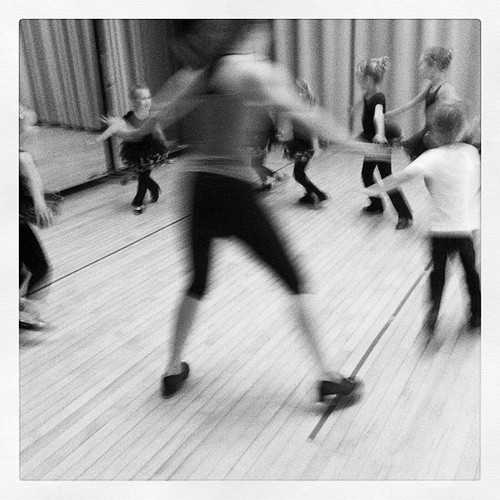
[19,104,55,331]
[357,56,414,230]
[246,107,282,193]
[385,47,471,159]
[92,83,169,212]
[363,98,481,331]
[283,79,329,205]
[116,19,406,403]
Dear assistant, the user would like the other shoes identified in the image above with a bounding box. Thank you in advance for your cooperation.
[364,205,383,213]
[162,361,189,399]
[19,311,46,329]
[396,215,409,229]
[319,377,358,396]
[318,193,326,200]
[150,185,159,202]
[134,206,141,214]
[299,194,316,204]
